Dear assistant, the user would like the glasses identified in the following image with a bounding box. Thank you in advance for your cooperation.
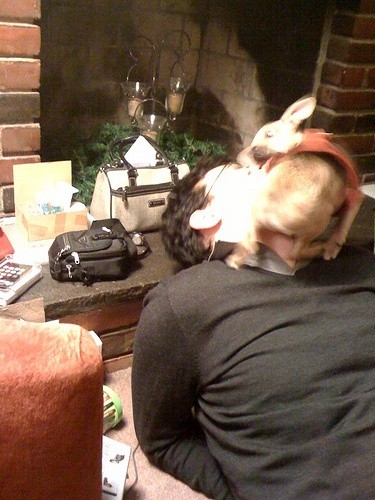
[198,161,244,212]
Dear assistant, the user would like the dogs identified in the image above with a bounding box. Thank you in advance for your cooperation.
[223,96,365,269]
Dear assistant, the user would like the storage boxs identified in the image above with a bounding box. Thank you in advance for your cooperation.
[14,161,88,242]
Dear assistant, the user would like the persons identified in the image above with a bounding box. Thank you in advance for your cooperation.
[131,162,375,500]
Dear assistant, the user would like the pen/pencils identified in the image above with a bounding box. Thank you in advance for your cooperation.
[103,385,123,435]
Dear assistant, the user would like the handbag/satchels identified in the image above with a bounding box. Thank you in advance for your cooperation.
[47,218,139,287]
[91,136,189,234]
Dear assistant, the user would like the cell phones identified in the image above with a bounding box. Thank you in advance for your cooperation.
[0,259,42,308]
